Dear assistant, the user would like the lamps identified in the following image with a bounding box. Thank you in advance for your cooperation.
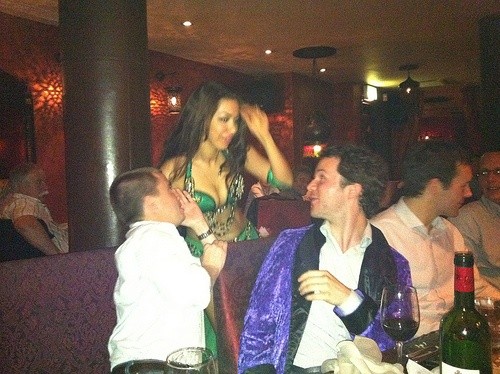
[156,70,182,114]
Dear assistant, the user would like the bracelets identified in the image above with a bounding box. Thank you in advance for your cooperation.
[198,229,213,240]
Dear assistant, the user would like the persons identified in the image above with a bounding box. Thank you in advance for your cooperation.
[244,164,313,228]
[369,139,500,342]
[107,167,228,374]
[156,80,293,374]
[446,146,500,291]
[237,142,412,374]
[0,160,68,262]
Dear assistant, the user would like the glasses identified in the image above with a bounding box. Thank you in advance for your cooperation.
[476,168,500,177]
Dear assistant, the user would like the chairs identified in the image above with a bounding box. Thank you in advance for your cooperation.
[213,235,278,374]
[0,246,118,374]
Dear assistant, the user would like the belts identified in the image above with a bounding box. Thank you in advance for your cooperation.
[304,366,321,374]
[112,359,198,374]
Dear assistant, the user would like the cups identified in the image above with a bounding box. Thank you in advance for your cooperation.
[160,347,218,374]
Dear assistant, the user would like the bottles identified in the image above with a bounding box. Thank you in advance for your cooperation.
[441,249,492,374]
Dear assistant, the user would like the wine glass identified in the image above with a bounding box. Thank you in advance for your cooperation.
[382,282,422,365]
